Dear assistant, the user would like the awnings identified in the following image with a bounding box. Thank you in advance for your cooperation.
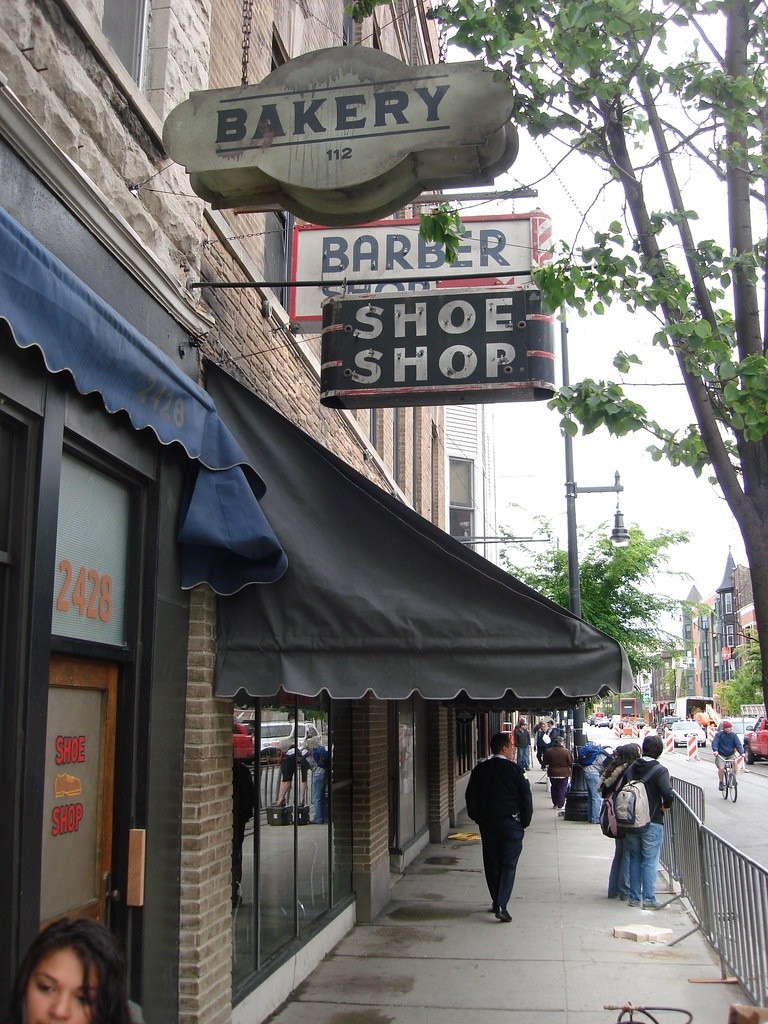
[197,348,637,713]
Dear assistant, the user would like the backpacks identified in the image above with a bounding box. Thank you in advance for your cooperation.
[600,778,624,838]
[613,763,663,834]
[578,743,613,766]
[300,745,328,769]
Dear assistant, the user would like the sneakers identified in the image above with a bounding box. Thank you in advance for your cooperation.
[495,905,513,922]
[492,902,496,912]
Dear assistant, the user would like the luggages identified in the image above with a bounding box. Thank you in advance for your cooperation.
[267,804,294,825]
[297,805,310,826]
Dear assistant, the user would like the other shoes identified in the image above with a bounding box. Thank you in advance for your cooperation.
[309,819,319,824]
[620,896,631,901]
[541,766,545,771]
[643,901,669,910]
[732,781,738,786]
[628,901,643,907]
[553,803,558,808]
[526,767,530,771]
[719,782,724,791]
[232,895,242,907]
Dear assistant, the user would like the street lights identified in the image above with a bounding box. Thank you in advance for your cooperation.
[558,296,632,820]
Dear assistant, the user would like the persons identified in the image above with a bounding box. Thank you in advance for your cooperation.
[299,747,328,824]
[543,737,573,811]
[230,761,254,908]
[533,719,558,770]
[513,716,531,774]
[579,736,674,910]
[7,916,136,1024]
[266,744,296,805]
[465,733,534,922]
[711,721,747,792]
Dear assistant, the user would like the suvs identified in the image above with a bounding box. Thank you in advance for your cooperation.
[238,720,321,763]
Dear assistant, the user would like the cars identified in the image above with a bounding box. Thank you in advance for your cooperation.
[588,713,620,729]
[657,716,684,739]
[637,718,647,730]
[670,721,707,748]
[743,715,768,765]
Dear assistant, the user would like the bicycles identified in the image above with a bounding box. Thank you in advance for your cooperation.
[714,752,745,803]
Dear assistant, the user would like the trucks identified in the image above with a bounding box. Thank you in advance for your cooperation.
[671,696,715,728]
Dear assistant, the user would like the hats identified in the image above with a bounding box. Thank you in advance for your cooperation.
[547,721,554,725]
[722,721,732,728]
[520,722,528,726]
